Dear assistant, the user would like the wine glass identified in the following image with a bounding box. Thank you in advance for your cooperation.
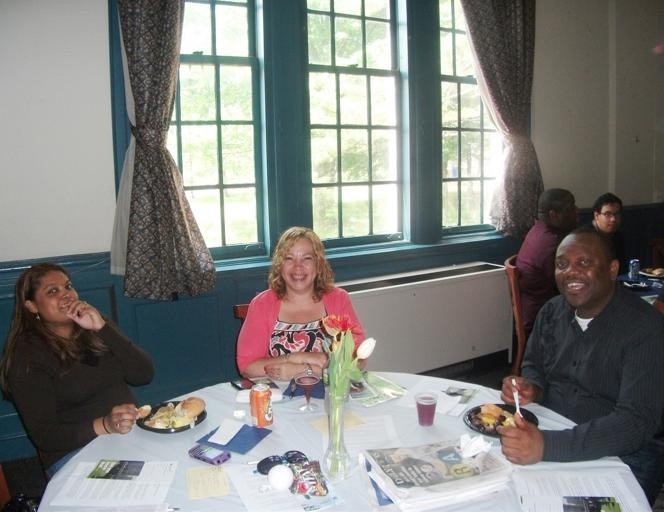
[294,372,320,412]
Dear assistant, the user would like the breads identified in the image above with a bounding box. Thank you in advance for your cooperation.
[183,396,204,415]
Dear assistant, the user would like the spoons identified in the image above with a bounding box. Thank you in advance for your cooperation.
[510,379,523,418]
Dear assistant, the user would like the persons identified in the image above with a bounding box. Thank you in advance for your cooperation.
[579,192,641,280]
[514,187,581,336]
[494,225,664,509]
[0,263,157,481]
[234,224,371,382]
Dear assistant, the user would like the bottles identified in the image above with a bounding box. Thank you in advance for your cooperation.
[249,384,272,427]
[629,259,640,280]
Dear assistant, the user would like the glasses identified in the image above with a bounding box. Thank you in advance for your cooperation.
[599,211,621,218]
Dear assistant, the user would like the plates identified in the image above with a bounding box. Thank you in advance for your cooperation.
[462,403,539,438]
[639,269,664,277]
[620,280,652,290]
[135,400,207,433]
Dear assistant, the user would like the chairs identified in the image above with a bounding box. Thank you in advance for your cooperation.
[654,299,664,315]
[504,252,532,377]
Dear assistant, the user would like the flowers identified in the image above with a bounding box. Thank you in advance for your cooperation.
[320,311,377,485]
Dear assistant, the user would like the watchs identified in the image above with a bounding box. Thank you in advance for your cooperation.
[305,363,315,378]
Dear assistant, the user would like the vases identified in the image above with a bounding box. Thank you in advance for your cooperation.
[321,396,353,483]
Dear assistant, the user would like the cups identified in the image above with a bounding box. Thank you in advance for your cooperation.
[415,392,437,426]
